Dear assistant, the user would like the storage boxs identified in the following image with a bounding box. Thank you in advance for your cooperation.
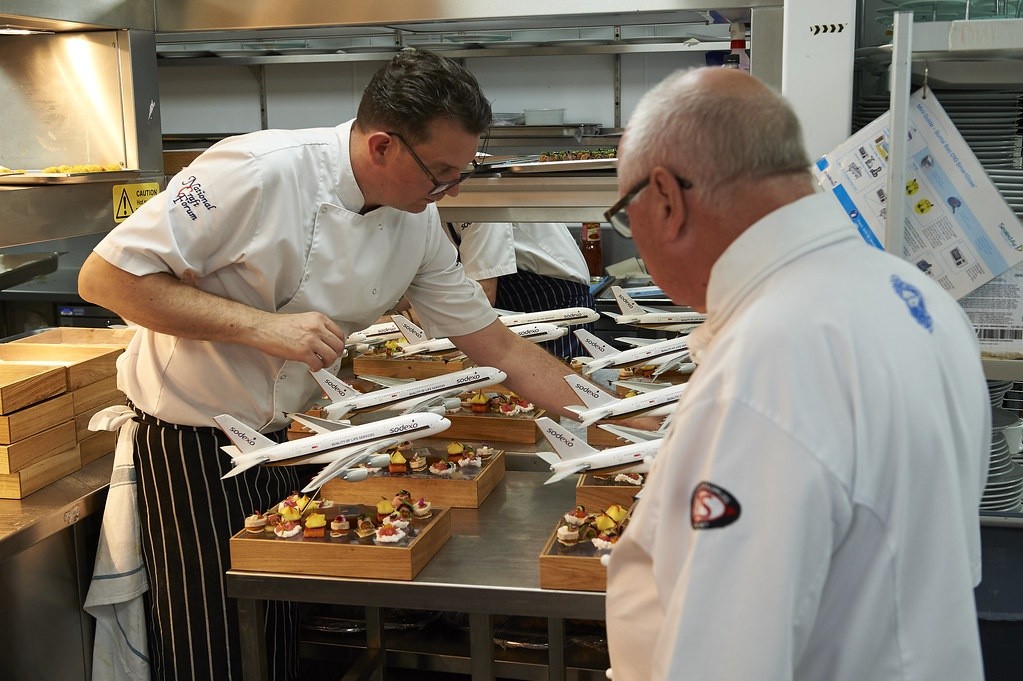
[0,327,135,500]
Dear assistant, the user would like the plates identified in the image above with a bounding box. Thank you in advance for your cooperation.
[979,379,1023,516]
[862,91,1023,223]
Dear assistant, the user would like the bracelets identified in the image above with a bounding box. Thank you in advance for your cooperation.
[394,309,413,322]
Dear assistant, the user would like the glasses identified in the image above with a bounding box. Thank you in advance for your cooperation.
[386,131,481,195]
[604,174,692,239]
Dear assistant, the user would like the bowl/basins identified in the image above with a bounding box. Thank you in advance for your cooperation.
[523,107,566,125]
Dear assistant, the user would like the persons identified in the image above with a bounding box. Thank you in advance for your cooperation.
[76,50,668,680]
[374,150,596,364]
[604,65,993,681]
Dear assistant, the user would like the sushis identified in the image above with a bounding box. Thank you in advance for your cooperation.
[244,339,644,549]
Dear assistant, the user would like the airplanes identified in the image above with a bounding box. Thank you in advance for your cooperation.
[571,286,707,383]
[531,413,668,488]
[305,361,509,426]
[211,409,453,516]
[390,312,565,364]
[493,301,601,336]
[563,373,691,433]
[339,321,402,359]
